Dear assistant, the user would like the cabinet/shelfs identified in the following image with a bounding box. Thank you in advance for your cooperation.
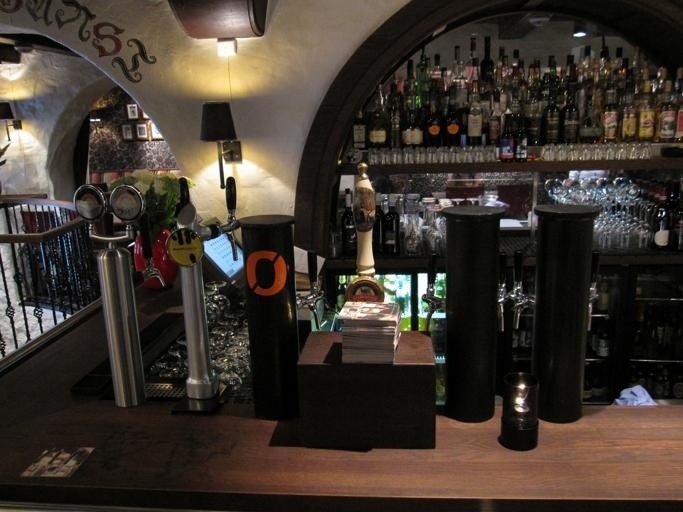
[331,153,681,400]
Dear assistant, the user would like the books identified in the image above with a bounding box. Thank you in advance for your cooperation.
[337,298,403,365]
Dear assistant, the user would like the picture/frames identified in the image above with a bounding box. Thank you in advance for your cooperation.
[118,98,164,144]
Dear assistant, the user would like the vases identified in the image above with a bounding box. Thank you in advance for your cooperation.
[129,228,177,292]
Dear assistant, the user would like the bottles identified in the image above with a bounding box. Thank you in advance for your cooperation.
[497,247,536,360]
[581,275,683,403]
[340,186,400,258]
[633,172,682,253]
[354,33,683,146]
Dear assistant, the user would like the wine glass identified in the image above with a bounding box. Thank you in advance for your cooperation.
[397,188,502,257]
[547,167,655,251]
[147,280,250,397]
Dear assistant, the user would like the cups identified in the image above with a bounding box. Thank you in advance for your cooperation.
[389,170,535,190]
[498,373,540,452]
[366,141,661,165]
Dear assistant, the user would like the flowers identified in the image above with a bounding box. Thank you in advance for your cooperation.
[107,163,189,260]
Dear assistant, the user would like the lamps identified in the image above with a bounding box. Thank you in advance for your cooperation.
[0,37,32,66]
[164,1,274,62]
[0,102,26,144]
[197,99,242,193]
